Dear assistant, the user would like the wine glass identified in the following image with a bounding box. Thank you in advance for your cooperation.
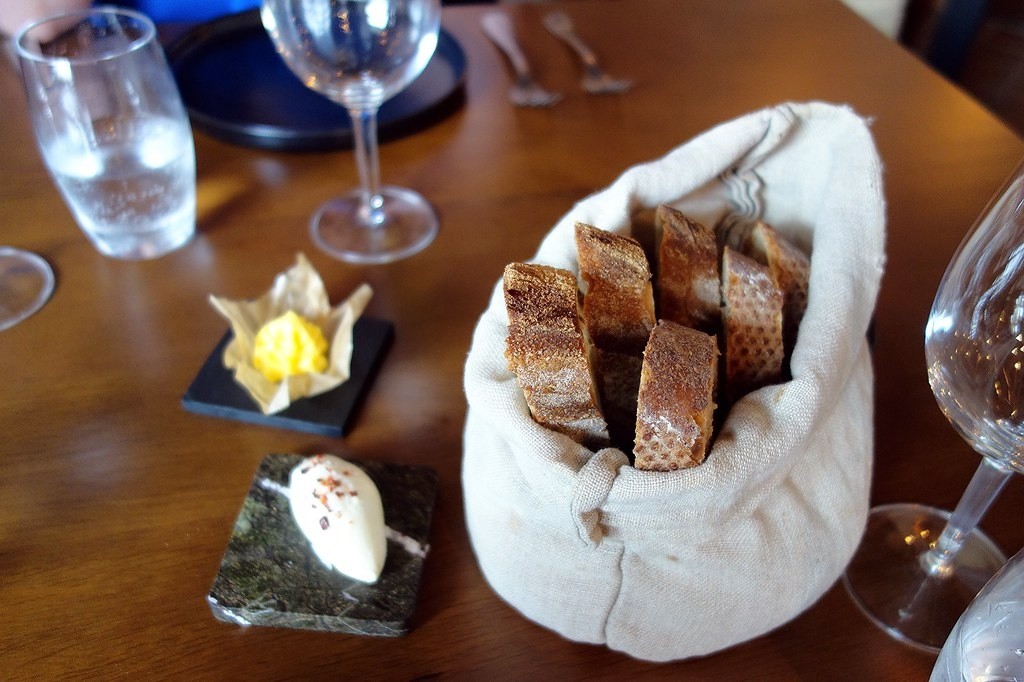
[844,158,1024,653]
[259,1,441,265]
[0,247,55,331]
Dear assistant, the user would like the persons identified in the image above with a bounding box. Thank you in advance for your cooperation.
[0,0,276,39]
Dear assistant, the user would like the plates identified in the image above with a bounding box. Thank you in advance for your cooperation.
[166,9,467,153]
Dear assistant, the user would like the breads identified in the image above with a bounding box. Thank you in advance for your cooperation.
[502,204,811,471]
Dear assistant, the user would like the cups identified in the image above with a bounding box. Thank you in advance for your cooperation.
[16,9,197,261]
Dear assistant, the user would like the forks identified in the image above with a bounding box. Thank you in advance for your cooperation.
[544,12,633,93]
[480,11,560,107]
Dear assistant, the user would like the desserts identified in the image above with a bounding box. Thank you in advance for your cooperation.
[209,253,375,415]
[287,452,386,583]
